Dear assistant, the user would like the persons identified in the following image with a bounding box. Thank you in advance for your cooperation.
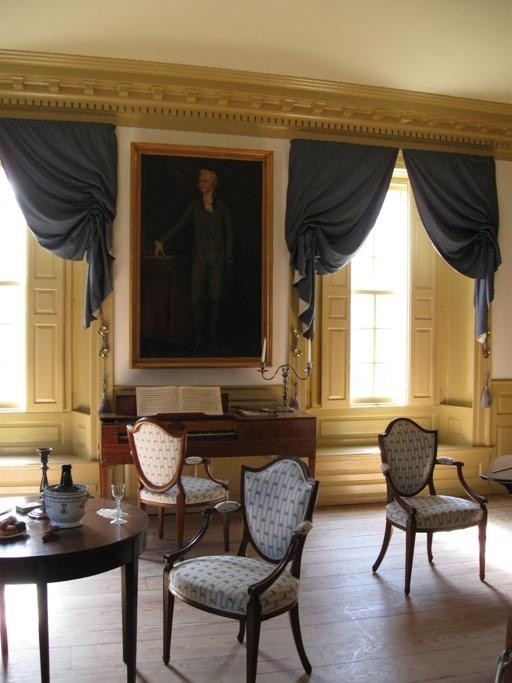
[155,166,234,353]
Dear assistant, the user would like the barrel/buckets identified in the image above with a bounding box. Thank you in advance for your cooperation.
[40,483,88,529]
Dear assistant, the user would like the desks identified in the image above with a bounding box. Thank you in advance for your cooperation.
[0,494,150,683]
[98,409,317,499]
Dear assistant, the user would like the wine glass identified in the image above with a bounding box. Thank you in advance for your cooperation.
[110,483,127,524]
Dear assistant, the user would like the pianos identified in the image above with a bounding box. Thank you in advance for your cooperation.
[98,385,316,498]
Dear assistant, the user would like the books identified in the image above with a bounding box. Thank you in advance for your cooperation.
[238,407,276,417]
[134,385,226,417]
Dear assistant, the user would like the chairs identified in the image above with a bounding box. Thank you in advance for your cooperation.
[372,417,488,595]
[162,453,319,683]
[125,416,230,561]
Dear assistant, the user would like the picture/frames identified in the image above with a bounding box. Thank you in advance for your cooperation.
[128,141,274,370]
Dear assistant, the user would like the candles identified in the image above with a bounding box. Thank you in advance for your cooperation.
[307,338,311,363]
[261,336,266,363]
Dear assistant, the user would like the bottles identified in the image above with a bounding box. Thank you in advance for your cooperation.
[50,463,81,493]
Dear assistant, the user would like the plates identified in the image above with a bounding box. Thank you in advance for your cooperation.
[0,525,30,539]
